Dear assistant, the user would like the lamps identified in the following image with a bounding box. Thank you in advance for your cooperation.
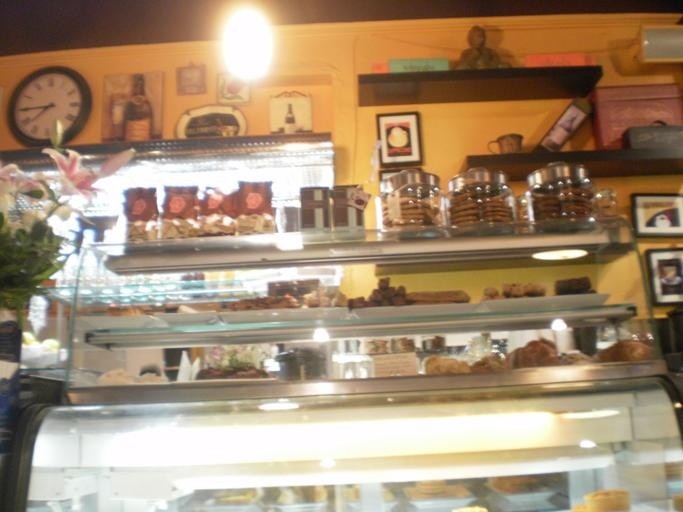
[611,21,683,75]
[216,5,277,84]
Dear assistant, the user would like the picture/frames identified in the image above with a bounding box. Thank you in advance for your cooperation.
[376,111,424,167]
[644,248,683,305]
[176,64,206,94]
[630,191,683,236]
[174,104,247,141]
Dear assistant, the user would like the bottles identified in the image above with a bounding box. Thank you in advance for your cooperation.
[283,102,298,134]
[124,74,153,143]
[274,347,313,380]
[375,160,620,241]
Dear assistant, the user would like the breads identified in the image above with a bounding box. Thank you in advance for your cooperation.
[424,338,655,375]
[227,278,599,312]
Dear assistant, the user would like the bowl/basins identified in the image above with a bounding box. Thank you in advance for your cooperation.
[423,336,446,353]
[390,339,416,353]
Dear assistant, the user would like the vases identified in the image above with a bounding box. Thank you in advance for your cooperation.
[0,291,21,442]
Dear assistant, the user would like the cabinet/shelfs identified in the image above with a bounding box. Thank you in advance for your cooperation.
[357,68,683,179]
[66,223,668,387]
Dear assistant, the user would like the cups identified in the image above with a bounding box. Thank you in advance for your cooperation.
[574,326,597,356]
[488,133,523,154]
[553,329,575,356]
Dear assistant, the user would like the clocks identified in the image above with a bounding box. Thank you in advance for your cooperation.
[7,64,91,145]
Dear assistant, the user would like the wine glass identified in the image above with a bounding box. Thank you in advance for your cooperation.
[108,91,124,141]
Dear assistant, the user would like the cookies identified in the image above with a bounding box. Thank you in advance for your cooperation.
[450,189,516,236]
[533,188,596,226]
[376,197,441,227]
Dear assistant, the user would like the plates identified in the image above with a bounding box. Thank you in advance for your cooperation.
[204,480,557,511]
[372,352,420,377]
[73,292,611,329]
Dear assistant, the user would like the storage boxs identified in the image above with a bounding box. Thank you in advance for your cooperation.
[591,82,683,151]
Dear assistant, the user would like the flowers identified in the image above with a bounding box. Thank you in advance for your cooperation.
[0,117,137,330]
